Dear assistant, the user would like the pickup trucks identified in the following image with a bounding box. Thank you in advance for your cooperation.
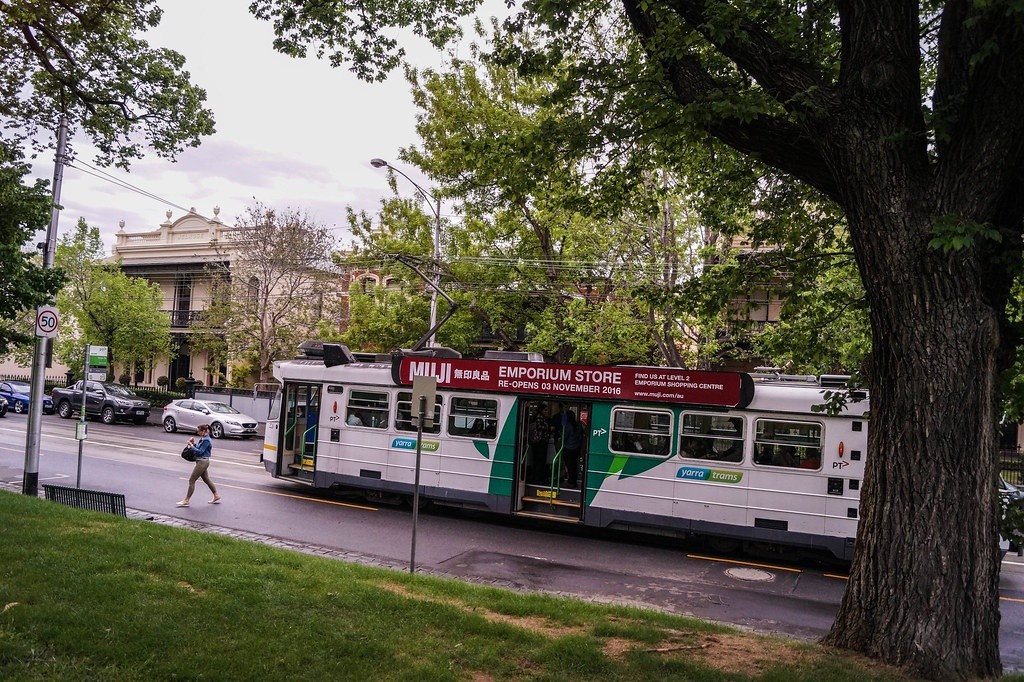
[51,380,152,424]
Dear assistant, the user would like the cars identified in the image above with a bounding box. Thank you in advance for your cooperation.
[162,400,259,439]
[0,396,8,418]
[0,379,55,416]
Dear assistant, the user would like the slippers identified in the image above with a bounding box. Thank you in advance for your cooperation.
[176,501,190,506]
[208,498,221,504]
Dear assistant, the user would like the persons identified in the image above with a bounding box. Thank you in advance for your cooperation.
[176,424,220,506]
[757,433,821,469]
[468,415,496,438]
[525,402,587,489]
[681,418,742,461]
[348,398,409,430]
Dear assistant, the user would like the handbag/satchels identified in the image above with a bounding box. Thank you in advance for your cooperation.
[181,443,196,462]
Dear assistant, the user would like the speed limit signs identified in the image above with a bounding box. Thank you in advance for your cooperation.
[38,311,59,333]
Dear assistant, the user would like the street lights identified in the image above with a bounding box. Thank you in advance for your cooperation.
[369,157,441,347]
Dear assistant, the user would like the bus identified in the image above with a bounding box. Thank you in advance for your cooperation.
[260,339,1024,568]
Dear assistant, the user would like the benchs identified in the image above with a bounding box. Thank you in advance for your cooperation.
[41,483,154,521]
